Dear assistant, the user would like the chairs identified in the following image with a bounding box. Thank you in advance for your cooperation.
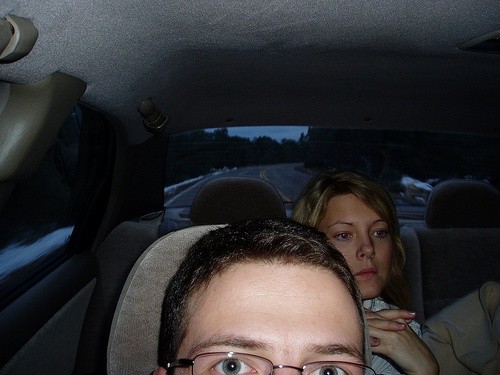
[402,180,500,324]
[72,174,288,374]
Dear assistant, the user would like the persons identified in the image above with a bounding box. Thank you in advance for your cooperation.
[152,215,381,375]
[291,172,440,375]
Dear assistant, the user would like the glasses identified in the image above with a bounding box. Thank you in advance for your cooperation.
[148,350,385,375]
